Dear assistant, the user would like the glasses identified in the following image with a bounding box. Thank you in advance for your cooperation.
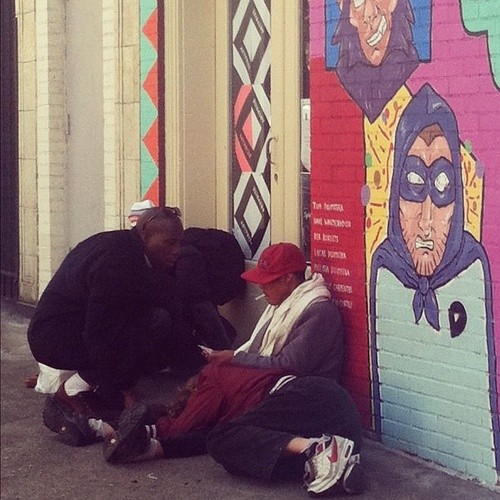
[141,206,181,231]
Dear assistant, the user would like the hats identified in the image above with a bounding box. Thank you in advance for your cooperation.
[241,242,307,285]
[128,199,155,217]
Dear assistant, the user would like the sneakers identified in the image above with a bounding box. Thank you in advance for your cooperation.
[43,394,94,441]
[299,434,354,497]
[103,407,151,463]
[52,380,105,444]
[343,454,377,494]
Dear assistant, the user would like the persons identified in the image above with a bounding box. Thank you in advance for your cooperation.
[128,200,238,375]
[103,243,348,463]
[26,206,184,447]
[125,361,366,494]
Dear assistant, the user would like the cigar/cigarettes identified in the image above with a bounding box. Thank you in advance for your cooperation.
[254,294,267,302]
